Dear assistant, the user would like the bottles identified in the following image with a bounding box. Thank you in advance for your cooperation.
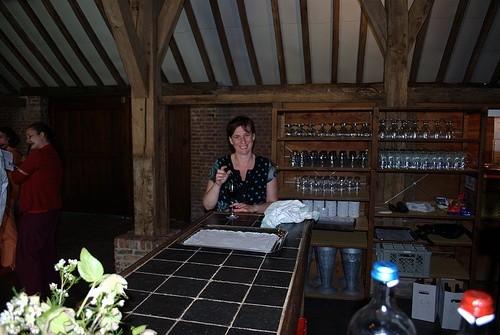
[349,260,416,335]
[457,290,495,335]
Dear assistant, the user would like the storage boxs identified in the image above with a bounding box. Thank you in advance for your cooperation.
[464,175,500,217]
[412,277,468,330]
[460,221,500,282]
[376,242,432,279]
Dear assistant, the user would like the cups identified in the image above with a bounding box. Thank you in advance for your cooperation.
[302,200,359,218]
[290,150,368,168]
[295,175,360,192]
[378,150,467,170]
[314,247,337,295]
[340,248,362,295]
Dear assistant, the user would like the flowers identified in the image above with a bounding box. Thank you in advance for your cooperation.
[0,248,158,335]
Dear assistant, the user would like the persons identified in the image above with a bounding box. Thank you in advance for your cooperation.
[203,115,278,214]
[0,122,63,302]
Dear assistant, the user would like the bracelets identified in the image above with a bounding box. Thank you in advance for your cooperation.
[215,182,221,187]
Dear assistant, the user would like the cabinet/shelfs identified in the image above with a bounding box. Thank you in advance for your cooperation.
[271,100,500,302]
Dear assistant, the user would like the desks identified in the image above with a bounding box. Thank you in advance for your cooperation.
[75,212,313,335]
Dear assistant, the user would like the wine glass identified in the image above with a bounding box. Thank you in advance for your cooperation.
[225,184,239,219]
[284,121,372,138]
[377,119,456,140]
